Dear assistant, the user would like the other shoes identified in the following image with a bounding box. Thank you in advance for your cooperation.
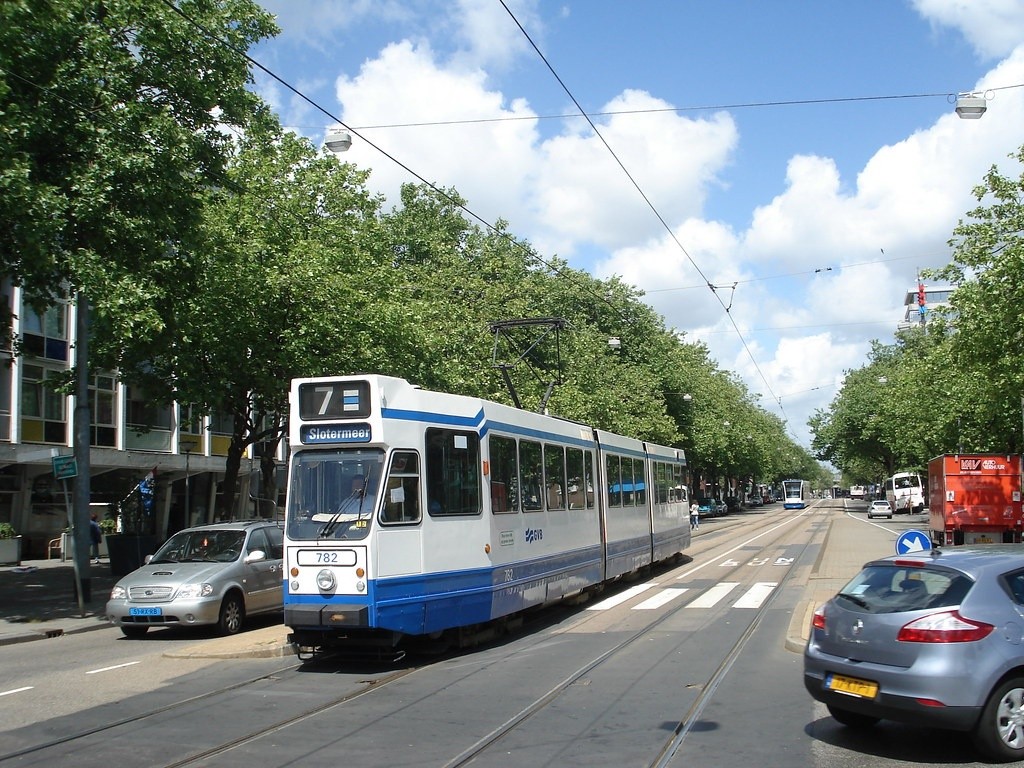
[696,528,699,530]
[691,528,694,530]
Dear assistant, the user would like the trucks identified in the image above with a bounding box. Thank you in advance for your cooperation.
[850,485,864,501]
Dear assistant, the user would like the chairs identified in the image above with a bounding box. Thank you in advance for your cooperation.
[898,579,927,595]
[46,536,61,560]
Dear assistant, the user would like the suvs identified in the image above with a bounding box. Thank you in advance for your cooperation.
[698,498,719,517]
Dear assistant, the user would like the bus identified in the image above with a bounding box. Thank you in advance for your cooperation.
[781,478,811,509]
[885,471,925,513]
[928,452,1023,549]
[282,375,691,663]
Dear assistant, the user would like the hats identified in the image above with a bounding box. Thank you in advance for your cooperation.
[692,500,697,504]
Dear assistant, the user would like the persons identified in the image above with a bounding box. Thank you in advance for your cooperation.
[90,513,102,563]
[336,475,386,522]
[690,500,699,531]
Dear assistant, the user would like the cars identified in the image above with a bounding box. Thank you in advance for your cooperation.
[715,500,729,516]
[764,494,782,503]
[103,517,287,637]
[802,540,1024,762]
[750,495,764,507]
[725,497,741,512]
[868,500,893,520]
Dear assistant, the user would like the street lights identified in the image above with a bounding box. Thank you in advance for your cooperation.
[179,440,197,530]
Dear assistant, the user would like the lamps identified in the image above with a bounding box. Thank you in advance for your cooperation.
[323,128,350,154]
[957,90,992,121]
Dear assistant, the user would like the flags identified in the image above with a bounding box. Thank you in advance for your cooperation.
[139,466,157,516]
